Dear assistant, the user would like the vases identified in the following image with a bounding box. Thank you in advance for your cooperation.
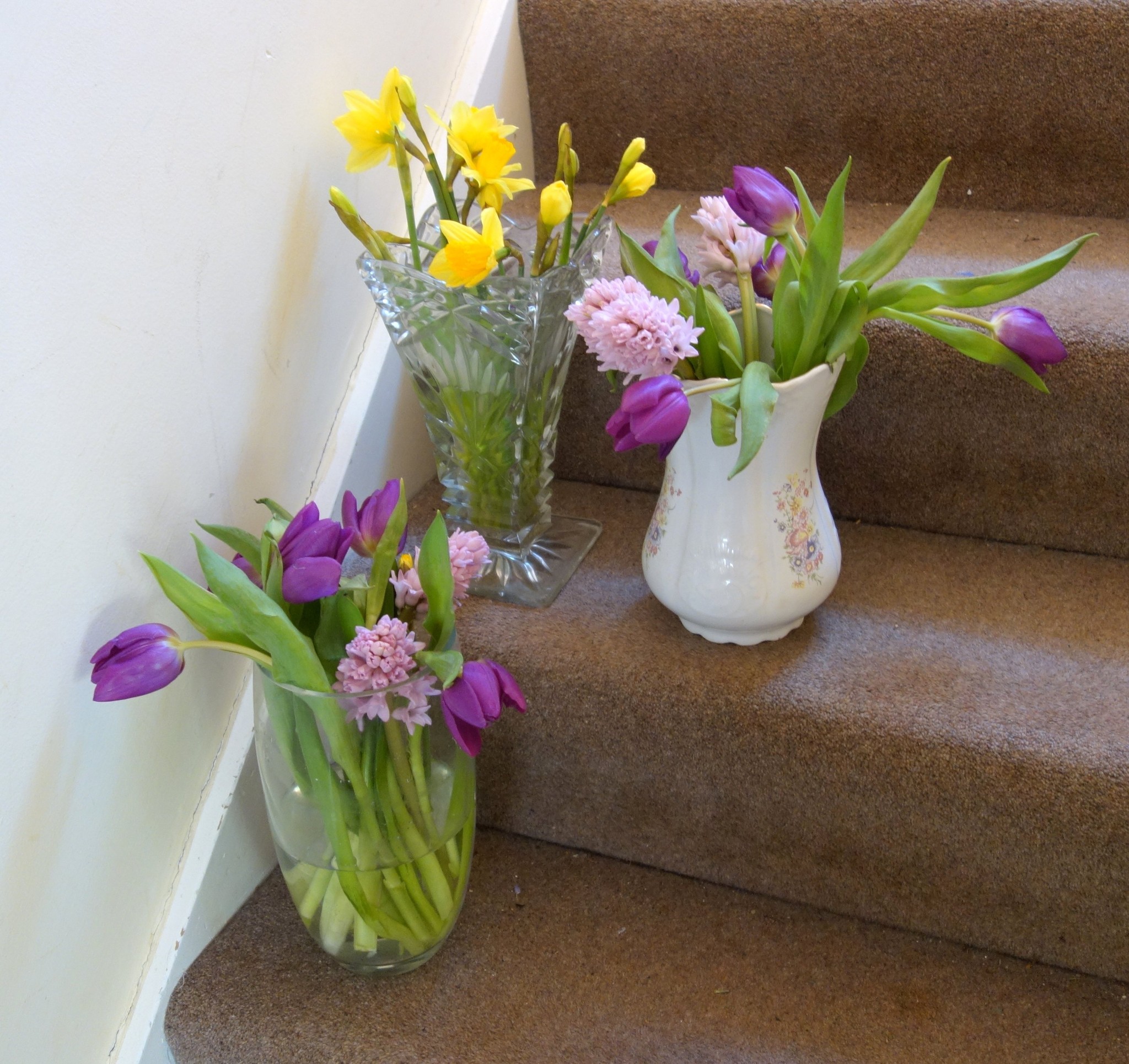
[357,199,611,607]
[642,303,845,646]
[253,632,477,978]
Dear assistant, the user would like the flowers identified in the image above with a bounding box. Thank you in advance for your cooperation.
[563,155,1100,478]
[90,477,530,961]
[329,66,658,526]
